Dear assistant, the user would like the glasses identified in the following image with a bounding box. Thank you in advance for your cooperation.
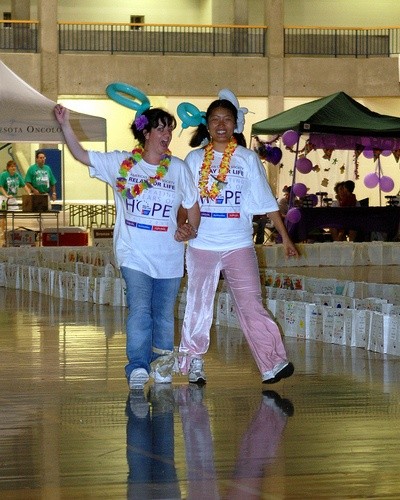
[37,157,46,160]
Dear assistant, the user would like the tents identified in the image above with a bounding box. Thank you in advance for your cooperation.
[249,90,400,216]
[0,60,108,236]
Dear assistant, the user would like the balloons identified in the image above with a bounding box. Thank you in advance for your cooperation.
[363,173,394,192]
[295,156,312,174]
[286,208,300,223]
[380,150,392,156]
[282,130,300,148]
[363,150,375,159]
[291,183,307,198]
[176,90,248,134]
[107,83,150,119]
[269,146,282,165]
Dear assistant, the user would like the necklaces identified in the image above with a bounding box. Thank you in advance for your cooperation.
[199,134,238,199]
[117,143,172,199]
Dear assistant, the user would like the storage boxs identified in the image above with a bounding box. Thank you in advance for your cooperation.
[21,194,49,213]
[42,226,89,246]
[6,227,36,245]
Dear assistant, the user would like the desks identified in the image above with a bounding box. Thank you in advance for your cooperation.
[7,210,59,248]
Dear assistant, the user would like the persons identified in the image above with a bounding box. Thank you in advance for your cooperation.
[124,390,294,500]
[331,180,359,242]
[175,99,301,385]
[25,151,57,210]
[275,185,298,217]
[0,161,31,201]
[53,103,201,389]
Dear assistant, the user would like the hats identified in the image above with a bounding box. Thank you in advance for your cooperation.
[286,186,296,198]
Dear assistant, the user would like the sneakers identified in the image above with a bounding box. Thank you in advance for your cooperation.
[186,382,207,402]
[261,360,295,385]
[128,368,149,390]
[129,390,148,419]
[261,390,295,417]
[187,358,207,383]
[149,363,174,383]
[149,383,172,403]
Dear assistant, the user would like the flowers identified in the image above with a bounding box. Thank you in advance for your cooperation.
[134,115,149,131]
